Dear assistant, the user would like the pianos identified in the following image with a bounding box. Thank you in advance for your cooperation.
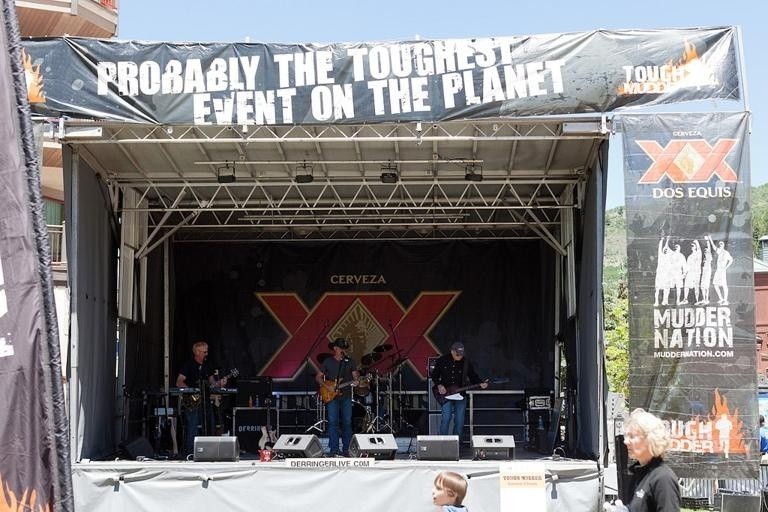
[169,387,237,397]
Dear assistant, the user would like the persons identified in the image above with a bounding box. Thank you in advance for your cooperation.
[653,232,734,307]
[176,341,228,460]
[314,338,361,457]
[604,406,682,511]
[759,415,768,452]
[431,340,488,453]
[431,471,470,512]
[715,413,733,459]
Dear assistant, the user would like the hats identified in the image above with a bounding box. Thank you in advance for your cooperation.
[328,338,349,350]
[450,342,464,356]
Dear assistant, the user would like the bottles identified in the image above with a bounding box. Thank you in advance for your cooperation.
[249,395,254,407]
[255,395,260,407]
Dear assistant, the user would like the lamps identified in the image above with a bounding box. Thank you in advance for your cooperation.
[216,166,486,183]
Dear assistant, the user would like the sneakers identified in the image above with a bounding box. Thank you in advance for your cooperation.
[342,449,350,457]
[329,450,338,458]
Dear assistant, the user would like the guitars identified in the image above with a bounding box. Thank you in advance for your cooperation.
[320,373,374,402]
[183,368,240,413]
[316,352,334,364]
[432,377,509,406]
[361,353,382,365]
[373,344,393,352]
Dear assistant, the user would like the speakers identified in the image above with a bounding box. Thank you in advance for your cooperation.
[193,436,240,461]
[428,412,455,435]
[117,435,154,461]
[416,434,460,461]
[615,434,638,505]
[348,433,398,460]
[272,434,325,457]
[471,434,515,461]
[428,378,456,412]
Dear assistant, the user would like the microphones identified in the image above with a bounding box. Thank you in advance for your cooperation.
[341,351,348,359]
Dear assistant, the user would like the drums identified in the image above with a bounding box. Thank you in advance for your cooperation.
[357,392,375,406]
[339,398,371,438]
